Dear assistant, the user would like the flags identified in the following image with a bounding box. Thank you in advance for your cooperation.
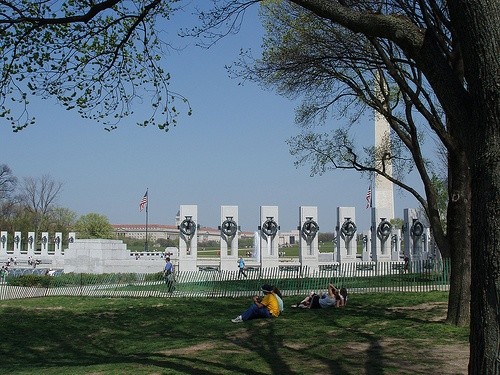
[140,192,147,212]
[366,186,371,208]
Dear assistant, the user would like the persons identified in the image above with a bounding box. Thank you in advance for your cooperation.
[164,258,176,293]
[291,283,348,310]
[237,256,247,279]
[271,286,284,314]
[230,285,279,323]
[404,254,410,273]
[0,257,55,277]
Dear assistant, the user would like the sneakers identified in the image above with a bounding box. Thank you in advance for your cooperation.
[232,315,243,322]
